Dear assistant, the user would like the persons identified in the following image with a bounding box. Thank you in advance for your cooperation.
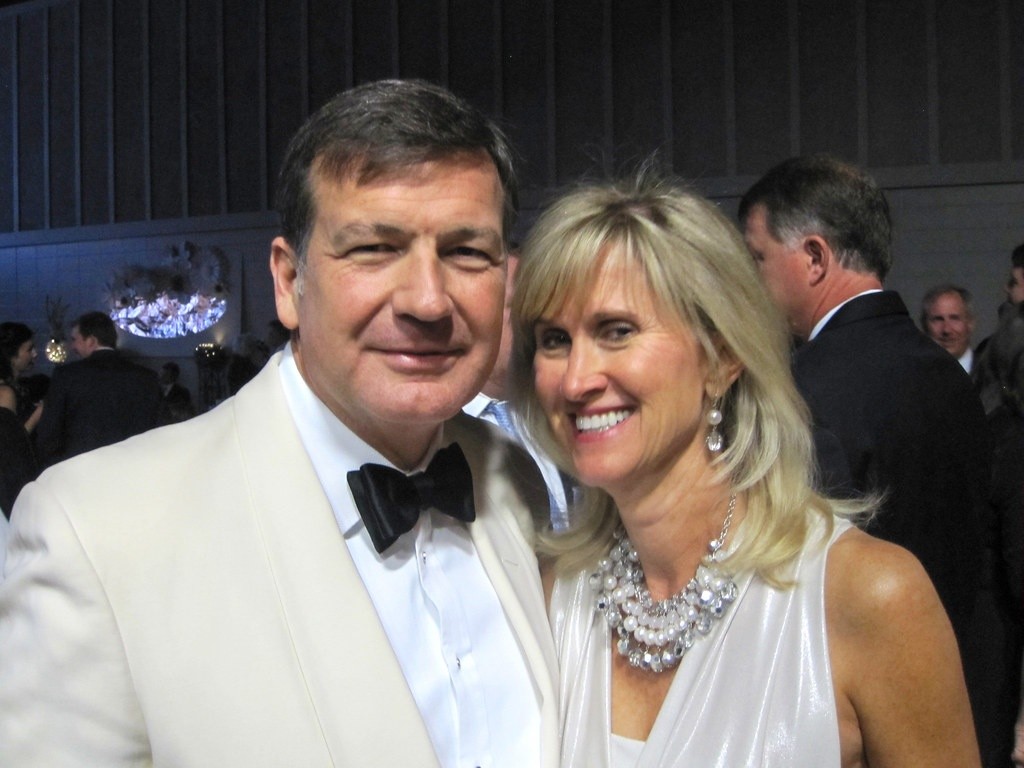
[1,79,1023,768]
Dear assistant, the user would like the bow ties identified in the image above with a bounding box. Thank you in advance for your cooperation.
[346,442,475,555]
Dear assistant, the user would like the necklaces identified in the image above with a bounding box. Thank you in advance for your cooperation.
[589,493,739,673]
